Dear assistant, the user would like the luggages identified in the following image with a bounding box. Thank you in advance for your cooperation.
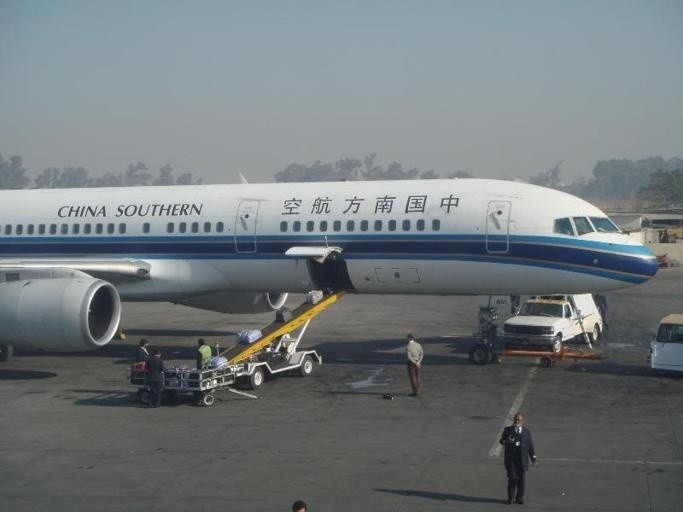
[207,356,228,372]
[131,362,149,370]
[165,368,180,386]
[236,329,263,346]
[189,370,213,387]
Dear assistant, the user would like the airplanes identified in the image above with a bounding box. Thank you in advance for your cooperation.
[1,176,659,365]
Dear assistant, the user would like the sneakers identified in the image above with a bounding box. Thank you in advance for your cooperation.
[508,497,524,504]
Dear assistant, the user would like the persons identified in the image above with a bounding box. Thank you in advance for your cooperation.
[292,499,307,512]
[405,333,425,398]
[196,338,212,368]
[137,339,150,363]
[499,413,538,504]
[511,294,520,315]
[145,350,164,408]
[593,291,609,332]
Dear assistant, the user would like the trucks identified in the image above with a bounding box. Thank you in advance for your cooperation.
[503,293,603,354]
